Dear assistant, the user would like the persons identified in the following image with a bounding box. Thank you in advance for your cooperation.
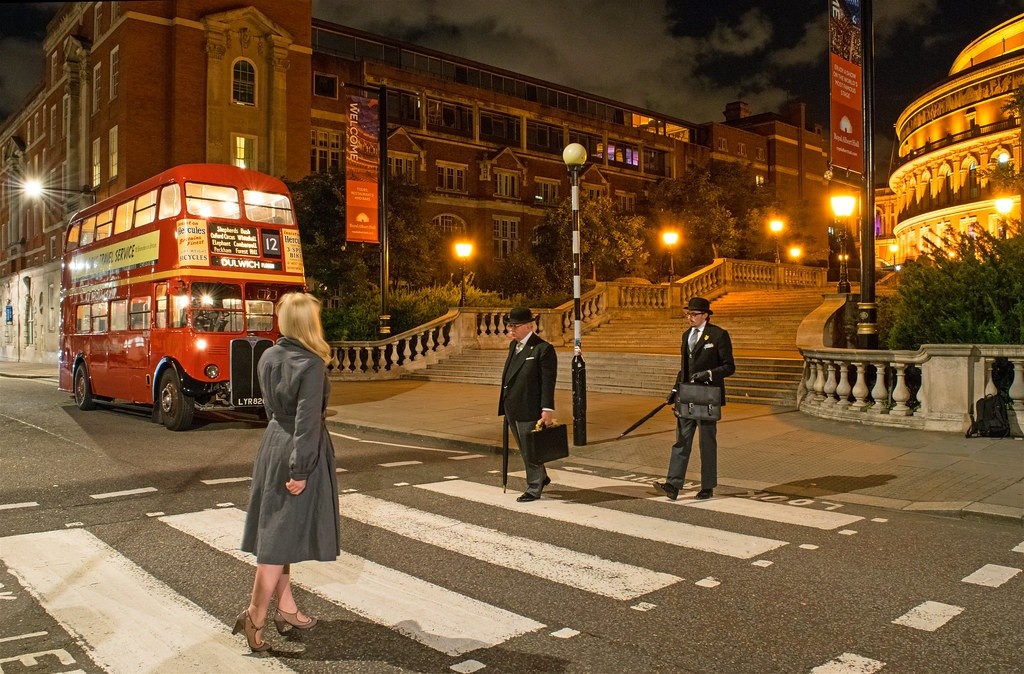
[232,291,340,652]
[653,297,736,501]
[498,308,558,502]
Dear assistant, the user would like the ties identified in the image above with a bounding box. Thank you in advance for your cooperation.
[514,342,523,352]
[687,328,701,352]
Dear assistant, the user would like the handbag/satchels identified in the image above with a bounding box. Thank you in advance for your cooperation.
[673,376,722,421]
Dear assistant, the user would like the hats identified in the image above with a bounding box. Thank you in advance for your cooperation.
[683,297,714,314]
[504,307,536,324]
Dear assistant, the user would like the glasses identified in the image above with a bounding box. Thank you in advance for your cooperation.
[685,312,706,316]
[507,322,526,329]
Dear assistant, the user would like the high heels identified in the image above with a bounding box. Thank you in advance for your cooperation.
[273,608,317,631]
[231,608,275,652]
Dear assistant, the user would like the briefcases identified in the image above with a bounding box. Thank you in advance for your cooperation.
[528,418,570,465]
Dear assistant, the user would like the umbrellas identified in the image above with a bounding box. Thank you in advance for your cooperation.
[616,402,667,440]
[503,416,509,492]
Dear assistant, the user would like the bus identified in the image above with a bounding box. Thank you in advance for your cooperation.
[58,163,308,432]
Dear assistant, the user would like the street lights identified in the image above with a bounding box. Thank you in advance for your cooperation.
[828,185,856,296]
[455,242,472,307]
[561,139,587,446]
[662,229,678,282]
[993,193,1015,240]
[769,219,785,265]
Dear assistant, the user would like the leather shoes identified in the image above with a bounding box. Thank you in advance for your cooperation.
[541,475,552,493]
[652,480,679,500]
[517,492,537,503]
[695,488,713,499]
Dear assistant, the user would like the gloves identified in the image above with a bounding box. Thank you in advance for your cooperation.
[691,371,710,382]
[666,391,676,405]
